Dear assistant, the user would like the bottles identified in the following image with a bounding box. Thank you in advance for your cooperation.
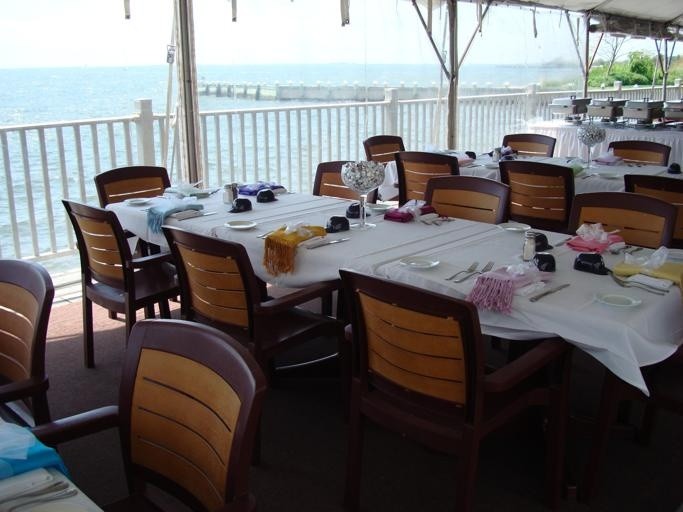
[492,147,501,163]
[222,182,238,205]
[521,230,537,262]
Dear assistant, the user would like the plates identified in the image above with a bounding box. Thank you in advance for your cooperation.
[595,290,642,308]
[483,163,500,169]
[400,255,439,269]
[367,202,393,211]
[497,222,532,232]
[224,220,257,231]
[125,197,153,205]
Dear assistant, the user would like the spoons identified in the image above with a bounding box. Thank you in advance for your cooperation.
[622,246,645,257]
[606,243,632,255]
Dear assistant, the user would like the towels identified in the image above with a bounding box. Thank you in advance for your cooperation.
[0,468,53,501]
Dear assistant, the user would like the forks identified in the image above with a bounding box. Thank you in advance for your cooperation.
[453,259,496,285]
[607,271,670,297]
[420,216,456,227]
[445,260,480,282]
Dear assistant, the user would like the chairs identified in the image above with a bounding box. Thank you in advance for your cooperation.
[93,164,188,325]
[332,266,574,510]
[0,257,55,425]
[29,307,272,510]
[298,99,683,250]
[158,222,350,469]
[60,199,195,370]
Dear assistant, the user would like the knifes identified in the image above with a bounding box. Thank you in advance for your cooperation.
[306,237,352,250]
[199,210,219,218]
[529,282,573,304]
[468,164,482,169]
[554,237,572,249]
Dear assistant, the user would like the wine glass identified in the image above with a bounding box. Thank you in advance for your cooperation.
[340,161,385,231]
[576,123,607,169]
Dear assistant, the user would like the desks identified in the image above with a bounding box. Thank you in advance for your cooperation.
[103,174,634,509]
